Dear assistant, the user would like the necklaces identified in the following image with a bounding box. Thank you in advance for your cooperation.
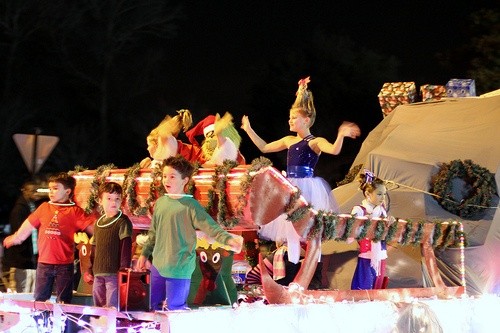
[164,193,194,198]
[48,201,76,206]
[97,210,123,228]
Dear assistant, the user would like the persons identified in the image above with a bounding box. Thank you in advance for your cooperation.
[83,183,133,311]
[4,172,96,303]
[241,76,360,290]
[147,109,245,166]
[7,178,39,294]
[347,170,396,290]
[137,156,241,310]
